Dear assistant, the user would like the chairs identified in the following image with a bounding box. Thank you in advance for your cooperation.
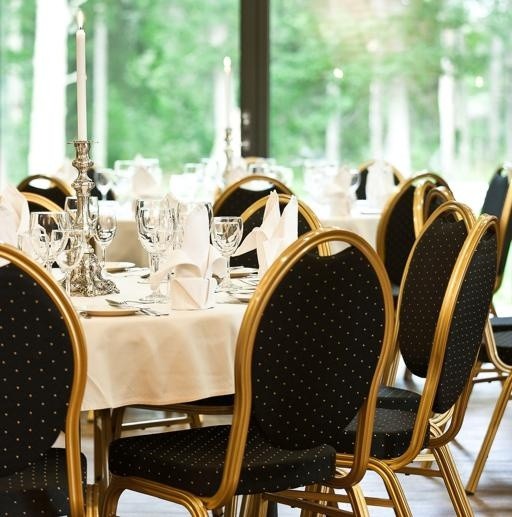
[0,156,512,517]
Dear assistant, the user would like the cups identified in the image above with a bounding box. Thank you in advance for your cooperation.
[63,196,100,247]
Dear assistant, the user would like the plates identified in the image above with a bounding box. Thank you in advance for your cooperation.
[71,298,143,318]
[230,266,260,278]
[98,259,135,274]
[211,287,264,303]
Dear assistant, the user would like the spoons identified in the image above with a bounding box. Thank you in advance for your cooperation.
[104,296,161,306]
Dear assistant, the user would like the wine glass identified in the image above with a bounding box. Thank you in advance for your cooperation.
[89,214,116,283]
[209,214,246,292]
[49,228,89,318]
[135,199,180,304]
[29,210,67,277]
